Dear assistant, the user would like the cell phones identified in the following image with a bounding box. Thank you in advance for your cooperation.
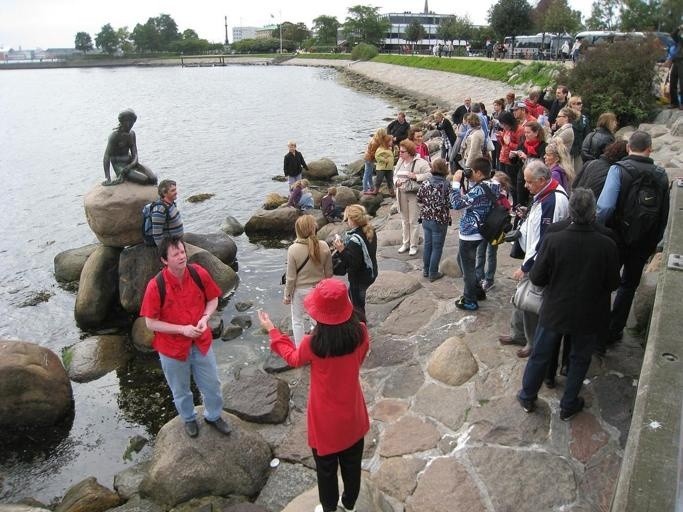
[514,203,526,217]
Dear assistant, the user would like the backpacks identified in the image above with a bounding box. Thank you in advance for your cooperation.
[614,160,665,248]
[472,182,513,246]
[142,202,169,246]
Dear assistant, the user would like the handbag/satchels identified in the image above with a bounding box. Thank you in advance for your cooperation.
[405,178,423,193]
[332,253,346,275]
[487,137,495,152]
[513,277,547,316]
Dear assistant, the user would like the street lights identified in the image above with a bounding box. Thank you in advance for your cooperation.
[270,16,282,54]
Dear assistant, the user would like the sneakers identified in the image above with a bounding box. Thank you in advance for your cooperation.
[455,278,496,311]
[423,271,444,283]
[498,336,584,421]
[315,495,357,512]
[398,243,419,256]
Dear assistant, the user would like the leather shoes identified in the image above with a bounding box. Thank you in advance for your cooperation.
[204,417,233,435]
[185,420,199,437]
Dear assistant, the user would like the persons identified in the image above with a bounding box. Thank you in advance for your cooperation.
[102,108,160,184]
[431,41,455,56]
[484,35,507,59]
[415,158,453,283]
[287,179,314,215]
[283,214,333,348]
[141,180,184,247]
[516,188,617,420]
[257,278,369,511]
[139,235,232,437]
[392,139,432,256]
[662,29,683,108]
[283,142,309,187]
[372,135,396,198]
[362,128,388,195]
[399,44,408,54]
[557,38,586,62]
[387,85,669,388]
[319,188,343,222]
[333,204,379,325]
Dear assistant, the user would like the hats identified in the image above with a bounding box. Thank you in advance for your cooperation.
[510,101,528,110]
[303,278,354,325]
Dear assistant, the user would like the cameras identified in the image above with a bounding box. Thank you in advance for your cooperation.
[546,89,550,98]
[335,234,343,241]
[463,169,472,178]
[510,151,519,157]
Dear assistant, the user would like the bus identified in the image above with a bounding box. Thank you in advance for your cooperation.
[500,30,675,60]
